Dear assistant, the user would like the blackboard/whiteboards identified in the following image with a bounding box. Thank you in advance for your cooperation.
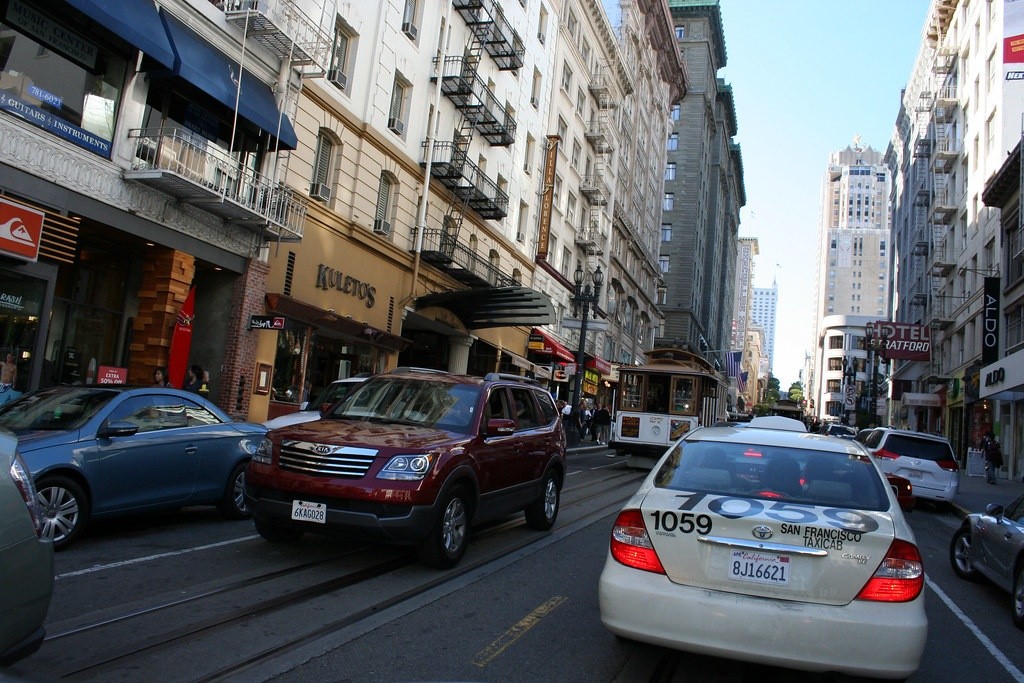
[966,451,986,476]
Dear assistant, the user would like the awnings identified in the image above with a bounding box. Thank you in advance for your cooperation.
[158,6,297,152]
[416,285,557,331]
[528,327,575,363]
[900,392,941,407]
[62,0,174,73]
[478,339,552,380]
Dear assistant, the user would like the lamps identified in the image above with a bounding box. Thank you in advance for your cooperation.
[957,261,999,278]
[374,219,391,235]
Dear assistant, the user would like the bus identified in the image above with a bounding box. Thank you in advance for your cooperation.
[609,347,730,469]
[767,398,802,422]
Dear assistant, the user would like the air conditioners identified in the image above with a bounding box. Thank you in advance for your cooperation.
[539,33,545,44]
[531,97,539,108]
[403,22,418,40]
[327,69,348,90]
[519,0,527,8]
[310,181,332,203]
[511,62,518,76]
[517,232,525,242]
[524,163,532,175]
[389,117,404,136]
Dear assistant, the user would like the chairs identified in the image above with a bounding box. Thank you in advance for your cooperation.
[808,480,853,501]
[683,467,732,490]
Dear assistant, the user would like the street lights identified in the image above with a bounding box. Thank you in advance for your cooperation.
[866,320,889,427]
[567,265,604,447]
[842,354,859,425]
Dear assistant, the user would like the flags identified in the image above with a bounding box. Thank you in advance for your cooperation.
[725,351,742,377]
[166,286,195,390]
[737,371,748,391]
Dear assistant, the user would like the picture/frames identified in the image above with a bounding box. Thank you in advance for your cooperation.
[253,361,273,396]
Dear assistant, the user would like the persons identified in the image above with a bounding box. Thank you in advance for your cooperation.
[556,399,611,446]
[0,353,17,392]
[150,367,174,389]
[183,364,208,397]
[980,433,1002,485]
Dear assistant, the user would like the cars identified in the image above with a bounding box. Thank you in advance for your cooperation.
[0,425,55,672]
[808,425,857,440]
[854,429,874,444]
[599,425,927,683]
[949,491,1024,630]
[0,383,269,552]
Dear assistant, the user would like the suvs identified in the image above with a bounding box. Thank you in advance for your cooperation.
[244,366,567,571]
[264,373,376,431]
[862,425,959,513]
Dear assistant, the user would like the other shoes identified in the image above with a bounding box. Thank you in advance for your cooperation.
[991,481,996,484]
[601,442,605,445]
[596,440,600,445]
[986,479,989,483]
